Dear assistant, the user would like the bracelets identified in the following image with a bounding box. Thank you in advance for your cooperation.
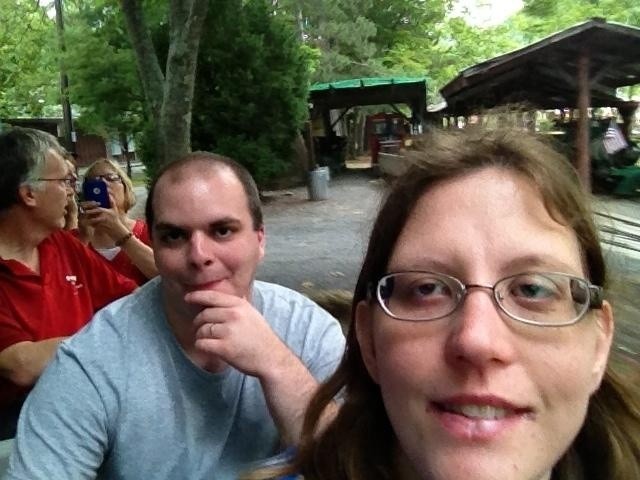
[116,230,132,247]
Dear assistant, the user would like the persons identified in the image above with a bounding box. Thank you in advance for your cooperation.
[73,157,158,287]
[3,150,348,480]
[247,115,639,480]
[0,126,140,480]
[64,156,79,231]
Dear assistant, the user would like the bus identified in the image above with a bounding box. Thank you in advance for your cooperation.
[365,112,409,174]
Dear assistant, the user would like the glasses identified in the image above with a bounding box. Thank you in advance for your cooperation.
[374,271,601,325]
[36,176,76,189]
[84,174,123,183]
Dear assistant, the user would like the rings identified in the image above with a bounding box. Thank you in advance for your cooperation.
[209,323,216,337]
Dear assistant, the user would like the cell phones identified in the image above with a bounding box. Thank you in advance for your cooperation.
[82,179,111,209]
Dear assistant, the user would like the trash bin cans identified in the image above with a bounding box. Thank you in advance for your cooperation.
[308,166,328,202]
